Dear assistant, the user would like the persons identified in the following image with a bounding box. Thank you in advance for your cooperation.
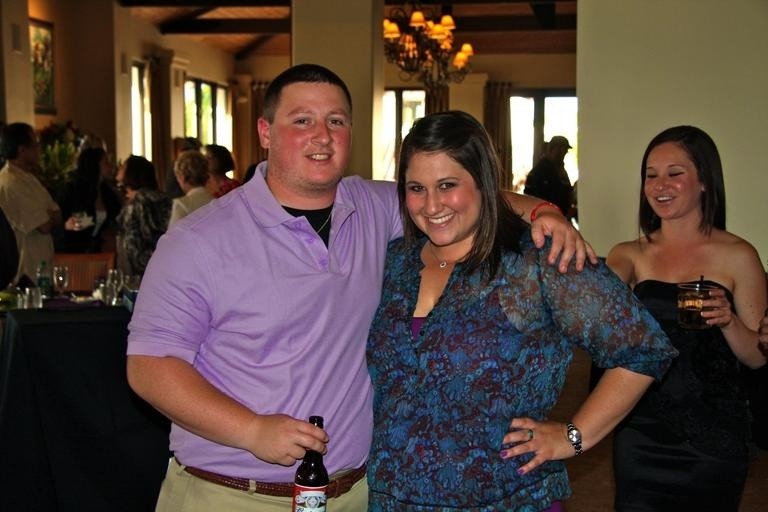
[0,120,266,331]
[524,135,578,221]
[125,63,599,511]
[756,307,768,360]
[360,109,680,512]
[600,125,768,510]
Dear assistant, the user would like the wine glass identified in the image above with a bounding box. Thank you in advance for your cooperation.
[105,268,122,306]
[51,266,70,299]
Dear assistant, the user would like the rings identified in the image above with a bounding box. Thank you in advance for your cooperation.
[527,428,534,441]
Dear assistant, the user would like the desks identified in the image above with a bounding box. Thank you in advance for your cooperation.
[1,290,105,314]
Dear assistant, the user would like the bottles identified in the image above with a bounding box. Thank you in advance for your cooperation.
[36,261,51,300]
[291,416,328,512]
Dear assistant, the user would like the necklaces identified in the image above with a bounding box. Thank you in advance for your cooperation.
[426,241,476,270]
[315,209,333,233]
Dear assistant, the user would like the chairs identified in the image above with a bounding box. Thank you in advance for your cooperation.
[1,307,170,512]
[47,252,116,289]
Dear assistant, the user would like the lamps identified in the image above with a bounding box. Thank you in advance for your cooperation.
[382,1,476,88]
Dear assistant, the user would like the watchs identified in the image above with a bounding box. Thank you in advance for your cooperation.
[564,418,585,457]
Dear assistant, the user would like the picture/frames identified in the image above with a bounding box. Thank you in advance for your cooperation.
[28,19,58,116]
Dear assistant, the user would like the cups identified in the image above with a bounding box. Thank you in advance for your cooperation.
[23,286,43,308]
[677,282,717,329]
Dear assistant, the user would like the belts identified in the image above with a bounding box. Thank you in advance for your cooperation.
[174,458,367,498]
